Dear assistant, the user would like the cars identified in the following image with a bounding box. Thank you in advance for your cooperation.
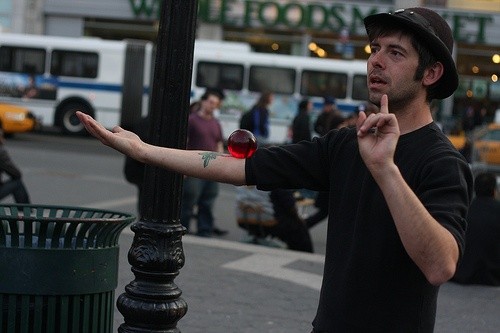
[447,108,500,166]
[0,102,37,140]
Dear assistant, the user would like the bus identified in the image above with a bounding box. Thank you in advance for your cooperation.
[0,33,372,151]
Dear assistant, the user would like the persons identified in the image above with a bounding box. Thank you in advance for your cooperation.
[291,96,316,143]
[20,74,38,101]
[77,6,477,332]
[180,83,228,239]
[237,88,273,148]
[314,92,347,136]
[340,102,379,130]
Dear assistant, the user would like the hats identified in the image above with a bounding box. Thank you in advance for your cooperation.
[364,7,458,99]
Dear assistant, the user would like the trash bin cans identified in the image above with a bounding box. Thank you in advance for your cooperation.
[0,204,136,333]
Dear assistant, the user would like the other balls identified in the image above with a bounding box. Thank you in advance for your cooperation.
[228,129,257,158]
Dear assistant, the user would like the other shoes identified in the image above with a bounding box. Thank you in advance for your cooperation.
[210,227,227,235]
